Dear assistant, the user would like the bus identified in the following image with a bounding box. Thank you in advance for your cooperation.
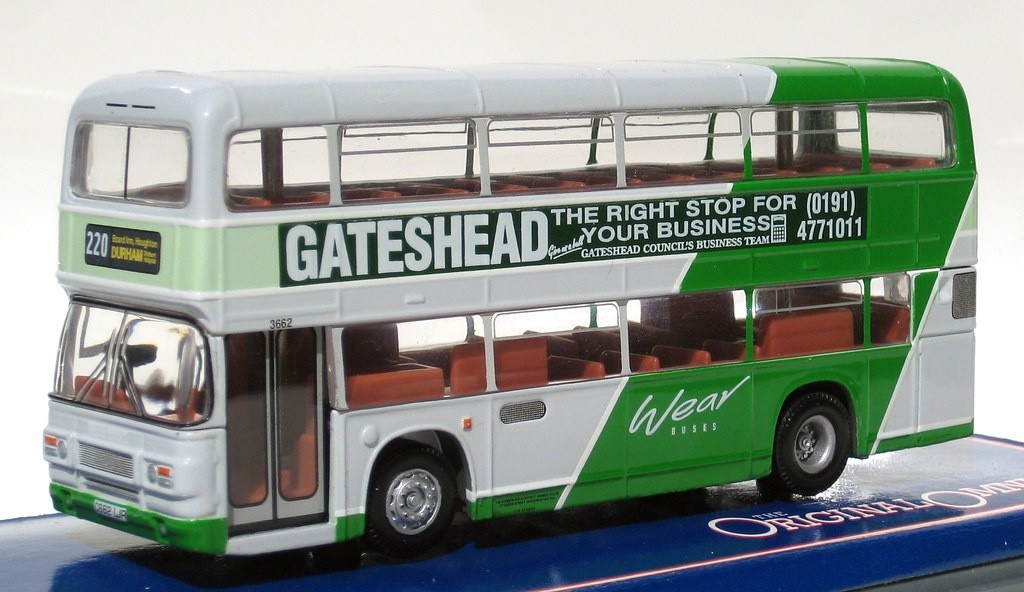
[26,53,983,567]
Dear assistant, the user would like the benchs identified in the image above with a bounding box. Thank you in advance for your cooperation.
[137,153,935,211]
[344,297,912,413]
